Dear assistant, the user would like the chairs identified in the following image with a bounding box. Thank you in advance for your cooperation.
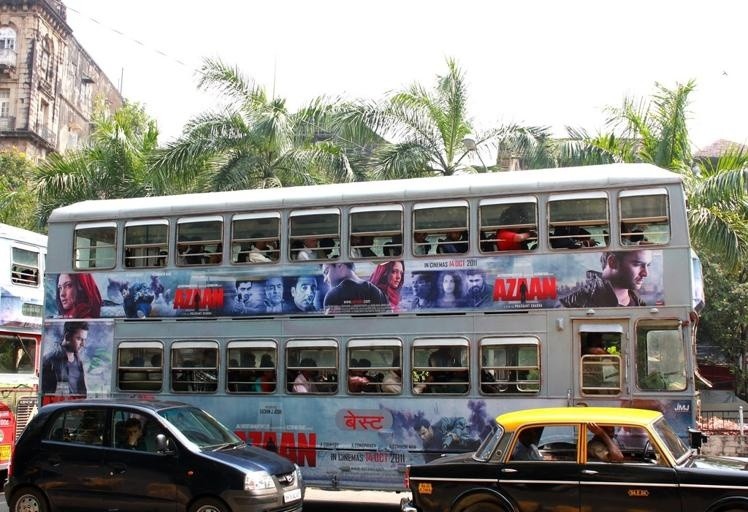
[374,373,382,392]
[234,380,247,390]
[603,230,612,247]
[145,423,161,451]
[383,241,396,256]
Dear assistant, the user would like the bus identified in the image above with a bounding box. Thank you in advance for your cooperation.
[39,162,707,495]
[0,219,47,470]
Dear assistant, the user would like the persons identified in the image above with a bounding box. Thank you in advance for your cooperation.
[416,416,482,463]
[58,252,653,320]
[120,355,501,395]
[581,332,610,394]
[514,428,545,461]
[154,203,654,266]
[42,321,89,394]
[599,426,620,448]
[117,417,147,451]
[586,422,624,462]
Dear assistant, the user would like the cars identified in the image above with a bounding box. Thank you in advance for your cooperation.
[2,398,305,512]
[401,405,748,511]
